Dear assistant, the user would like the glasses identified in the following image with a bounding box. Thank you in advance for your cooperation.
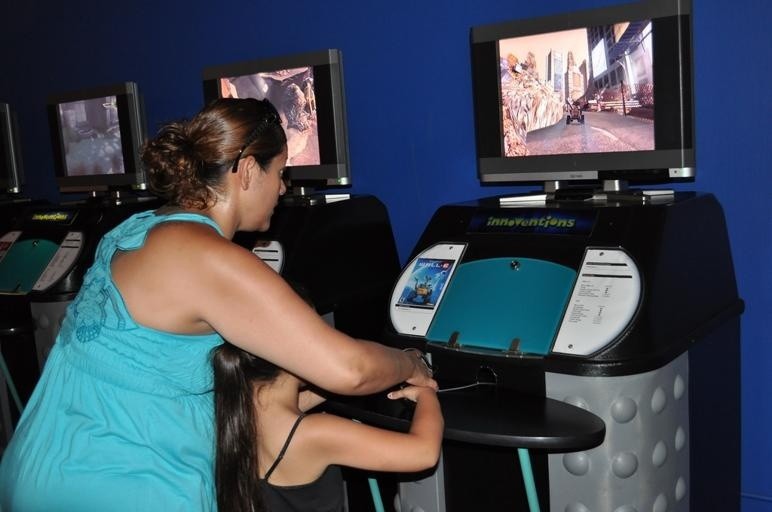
[232,98,283,173]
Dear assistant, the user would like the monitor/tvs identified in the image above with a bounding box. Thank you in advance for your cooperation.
[44,81,153,204]
[0,102,30,201]
[469,0,695,204]
[199,47,352,204]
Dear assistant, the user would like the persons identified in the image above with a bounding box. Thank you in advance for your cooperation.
[212,341,444,512]
[1,98,440,512]
